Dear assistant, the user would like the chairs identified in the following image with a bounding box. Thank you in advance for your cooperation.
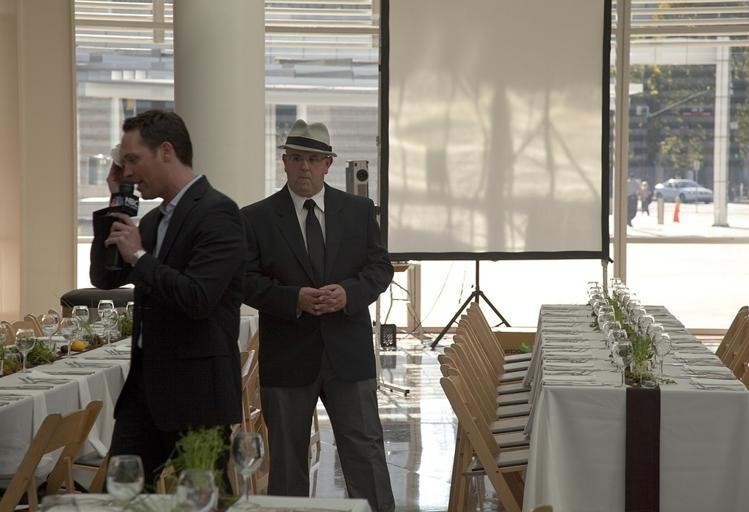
[150,326,317,496]
[0,400,100,512]
[437,302,533,512]
[46,441,112,493]
[713,305,749,390]
[1,307,66,372]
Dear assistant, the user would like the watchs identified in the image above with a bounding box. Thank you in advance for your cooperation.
[132,249,147,267]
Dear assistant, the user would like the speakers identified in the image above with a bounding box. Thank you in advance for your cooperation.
[346,160,370,198]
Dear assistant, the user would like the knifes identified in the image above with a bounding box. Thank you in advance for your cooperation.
[542,304,589,385]
[666,328,743,390]
[0,383,54,391]
[0,393,32,396]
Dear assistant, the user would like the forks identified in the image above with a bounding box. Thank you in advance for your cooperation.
[18,376,72,384]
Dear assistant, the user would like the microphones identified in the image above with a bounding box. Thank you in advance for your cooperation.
[106,181,139,269]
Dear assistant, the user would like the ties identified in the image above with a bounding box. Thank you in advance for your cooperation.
[303,199,326,288]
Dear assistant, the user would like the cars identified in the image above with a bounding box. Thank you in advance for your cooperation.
[653,178,714,204]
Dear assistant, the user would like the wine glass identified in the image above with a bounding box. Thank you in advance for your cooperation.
[587,277,672,387]
[230,431,264,509]
[0,299,135,377]
[106,454,144,512]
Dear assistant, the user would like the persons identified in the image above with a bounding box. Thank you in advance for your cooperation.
[241,120,396,512]
[640,181,654,216]
[627,173,643,227]
[90,108,248,494]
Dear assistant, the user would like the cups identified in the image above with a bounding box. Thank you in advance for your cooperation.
[176,468,215,512]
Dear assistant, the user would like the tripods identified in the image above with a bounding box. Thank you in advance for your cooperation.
[430,261,511,348]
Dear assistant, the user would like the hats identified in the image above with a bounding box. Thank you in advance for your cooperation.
[277,119,337,156]
